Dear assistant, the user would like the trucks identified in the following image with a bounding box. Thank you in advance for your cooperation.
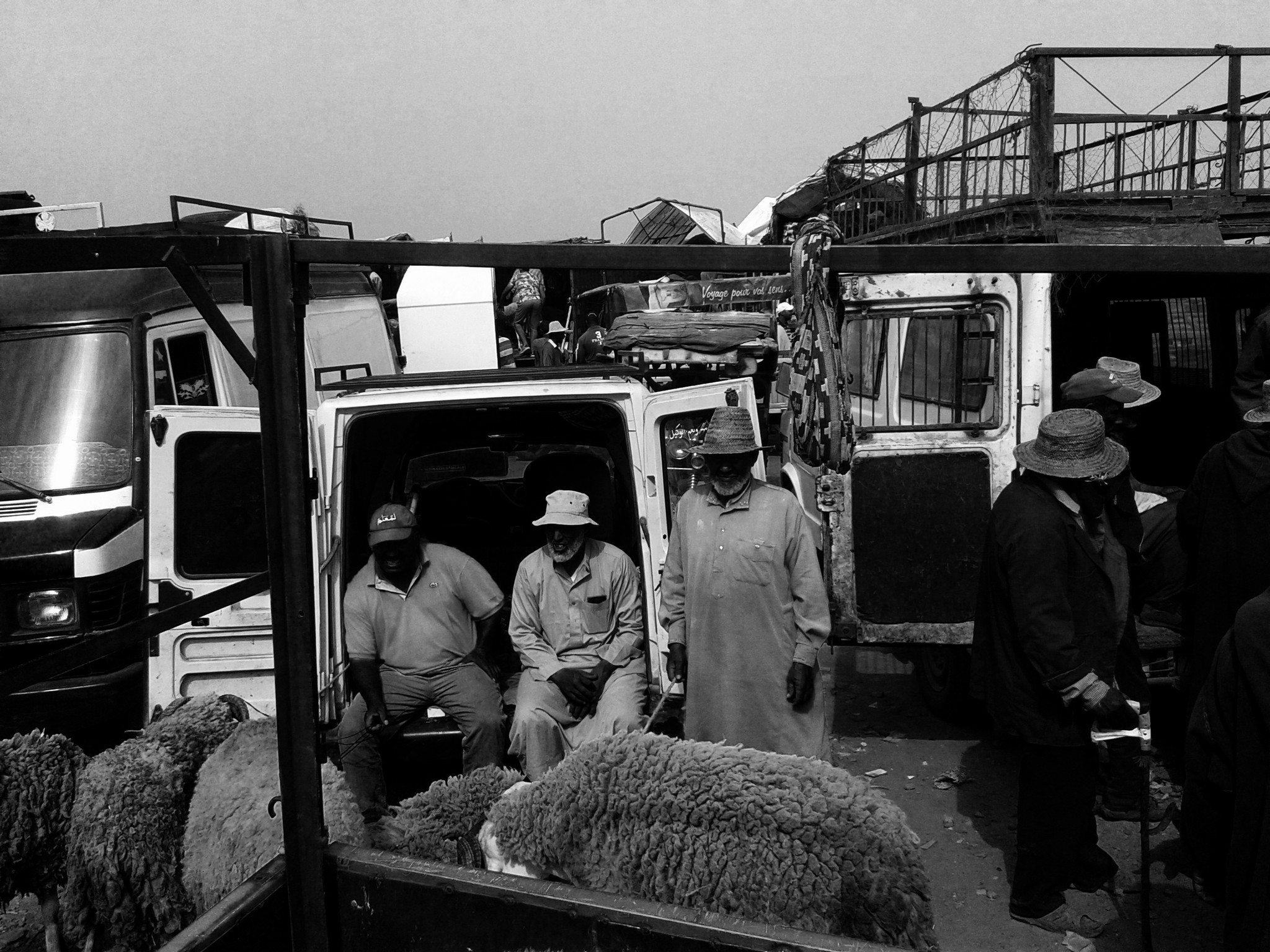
[0,247,1270,826]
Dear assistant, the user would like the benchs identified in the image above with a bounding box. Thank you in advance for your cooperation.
[415,452,612,571]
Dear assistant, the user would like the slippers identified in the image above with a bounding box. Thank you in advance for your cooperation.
[1071,868,1149,893]
[1009,903,1103,938]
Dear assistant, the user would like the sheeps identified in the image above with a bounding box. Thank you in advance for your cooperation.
[0,685,939,951]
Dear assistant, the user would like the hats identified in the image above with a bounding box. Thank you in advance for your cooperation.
[531,490,599,527]
[683,407,775,456]
[1082,355,1161,406]
[1061,367,1145,405]
[1013,408,1130,482]
[1243,379,1270,422]
[369,503,417,545]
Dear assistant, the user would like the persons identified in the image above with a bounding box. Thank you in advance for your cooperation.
[510,488,650,776]
[868,210,894,230]
[977,405,1140,934]
[541,320,568,366]
[659,406,833,761]
[1062,311,1270,951]
[337,502,510,826]
[533,322,549,368]
[576,310,614,362]
[496,263,546,357]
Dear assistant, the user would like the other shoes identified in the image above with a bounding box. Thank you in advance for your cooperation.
[1098,794,1167,821]
